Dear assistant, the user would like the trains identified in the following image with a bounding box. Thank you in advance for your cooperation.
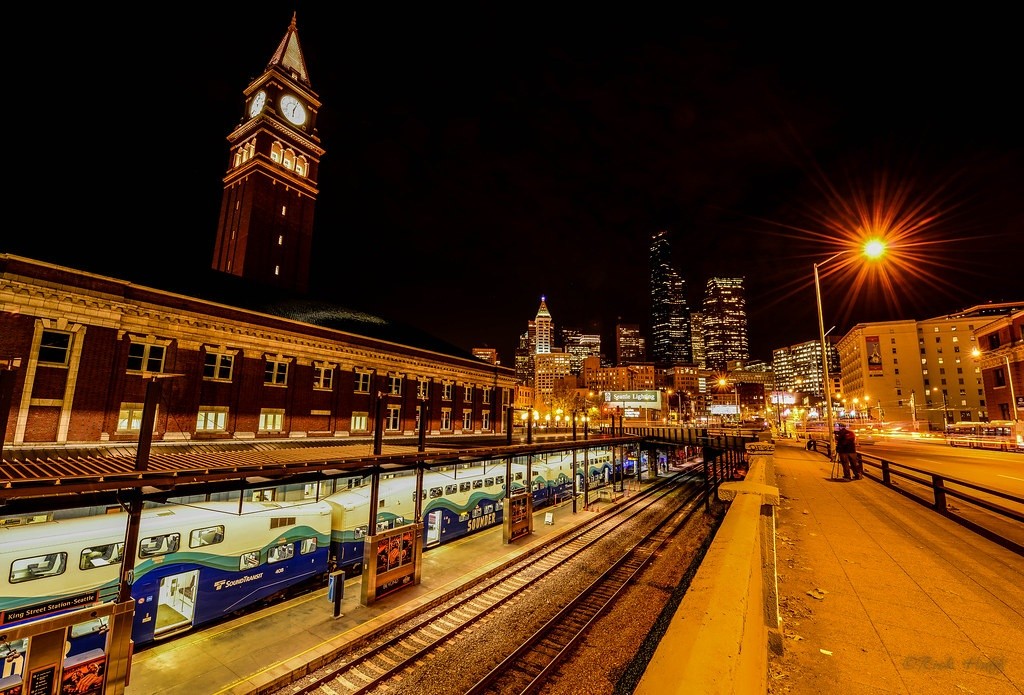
[0,451,622,672]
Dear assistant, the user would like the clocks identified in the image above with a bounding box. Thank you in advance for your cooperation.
[280,95,306,125]
[249,91,266,118]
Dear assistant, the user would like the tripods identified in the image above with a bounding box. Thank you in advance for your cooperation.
[831,452,840,478]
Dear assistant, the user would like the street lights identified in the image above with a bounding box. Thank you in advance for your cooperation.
[555,415,560,434]
[545,414,550,434]
[973,349,1017,420]
[667,389,681,422]
[813,240,889,461]
[520,413,528,435]
[780,379,803,437]
[720,379,739,436]
[565,415,570,433]
[533,411,539,434]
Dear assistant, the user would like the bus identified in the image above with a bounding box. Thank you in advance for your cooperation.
[796,419,850,439]
[949,421,1024,453]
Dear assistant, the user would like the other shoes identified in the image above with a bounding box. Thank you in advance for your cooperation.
[842,476,851,479]
[852,476,859,478]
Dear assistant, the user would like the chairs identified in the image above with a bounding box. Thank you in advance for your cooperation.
[27,553,66,579]
[272,544,294,560]
[212,533,223,544]
[142,536,180,557]
[101,544,120,562]
[300,540,316,553]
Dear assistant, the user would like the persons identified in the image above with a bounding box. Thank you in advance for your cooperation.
[832,422,863,481]
[62,662,104,695]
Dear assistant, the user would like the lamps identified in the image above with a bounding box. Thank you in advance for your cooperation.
[91,611,110,635]
[0,635,20,662]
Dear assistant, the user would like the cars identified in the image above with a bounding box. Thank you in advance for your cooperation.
[856,434,875,445]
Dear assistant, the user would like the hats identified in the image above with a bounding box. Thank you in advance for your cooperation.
[839,424,846,427]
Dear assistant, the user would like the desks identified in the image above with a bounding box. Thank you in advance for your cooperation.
[90,558,110,567]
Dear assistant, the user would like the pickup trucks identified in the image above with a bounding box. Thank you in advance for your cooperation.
[724,422,737,428]
